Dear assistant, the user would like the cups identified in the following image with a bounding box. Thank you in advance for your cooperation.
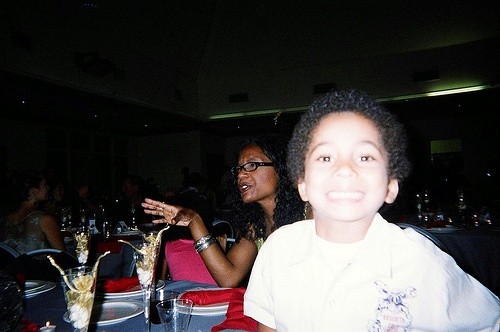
[60,204,110,237]
[156,299,193,332]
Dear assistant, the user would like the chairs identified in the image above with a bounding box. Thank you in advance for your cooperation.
[0,235,236,286]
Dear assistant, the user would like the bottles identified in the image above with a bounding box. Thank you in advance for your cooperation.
[456,186,469,227]
[415,186,435,226]
[128,200,138,230]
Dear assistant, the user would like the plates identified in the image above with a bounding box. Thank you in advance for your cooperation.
[96,278,166,299]
[121,231,140,233]
[22,280,56,298]
[171,288,232,317]
[63,299,144,326]
[420,224,466,233]
[113,233,140,235]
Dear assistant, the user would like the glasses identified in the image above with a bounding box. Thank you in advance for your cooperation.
[230,161,274,176]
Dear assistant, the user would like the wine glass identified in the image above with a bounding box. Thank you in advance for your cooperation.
[133,244,160,332]
[60,266,98,332]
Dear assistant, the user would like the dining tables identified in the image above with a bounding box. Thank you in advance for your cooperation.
[59,228,169,254]
[0,289,264,332]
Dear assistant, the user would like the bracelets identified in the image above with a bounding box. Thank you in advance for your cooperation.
[193,233,217,254]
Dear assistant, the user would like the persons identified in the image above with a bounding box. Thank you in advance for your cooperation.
[242,92,500,332]
[0,165,73,260]
[34,164,222,230]
[378,154,500,223]
[141,139,315,289]
